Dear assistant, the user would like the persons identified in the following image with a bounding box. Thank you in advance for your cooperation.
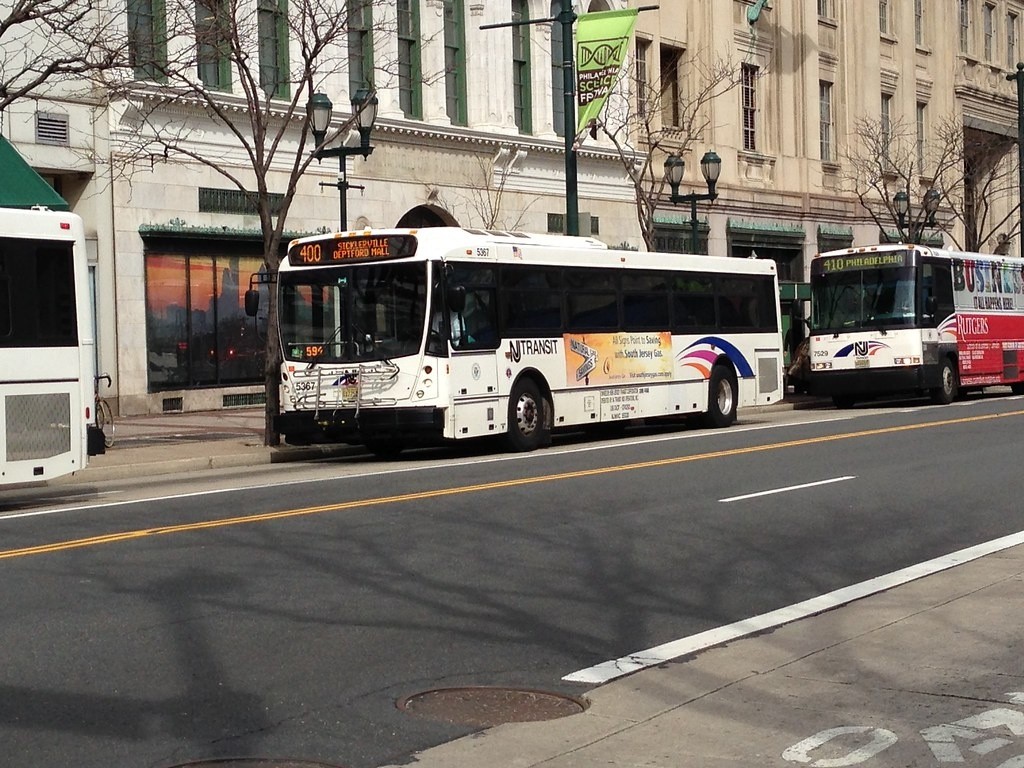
[430,292,466,346]
[784,320,805,363]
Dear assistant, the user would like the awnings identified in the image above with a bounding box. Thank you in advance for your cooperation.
[0,132,69,212]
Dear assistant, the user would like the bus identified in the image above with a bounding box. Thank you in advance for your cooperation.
[792,243,1024,403]
[244,226,783,455]
[0,207,86,489]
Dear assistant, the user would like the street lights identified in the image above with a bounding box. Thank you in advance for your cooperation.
[893,189,940,245]
[664,150,722,255]
[306,87,379,231]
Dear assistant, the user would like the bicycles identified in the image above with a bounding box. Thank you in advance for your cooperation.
[94,372,115,448]
